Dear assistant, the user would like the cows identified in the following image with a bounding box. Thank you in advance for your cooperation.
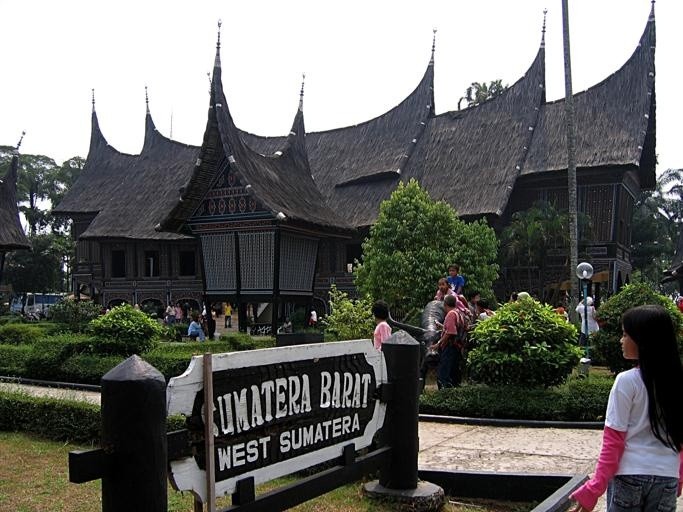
[387,299,481,393]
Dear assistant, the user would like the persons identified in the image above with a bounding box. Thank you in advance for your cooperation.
[574,296,600,347]
[44,304,52,321]
[565,303,682,512]
[279,317,293,334]
[428,264,533,389]
[99,301,158,321]
[370,299,392,352]
[164,301,216,341]
[223,301,232,328]
[554,302,564,320]
[308,308,317,326]
[563,305,569,323]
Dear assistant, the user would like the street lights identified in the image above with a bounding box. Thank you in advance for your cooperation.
[575,262,593,379]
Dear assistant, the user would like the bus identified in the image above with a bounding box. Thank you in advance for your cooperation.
[9,291,73,320]
